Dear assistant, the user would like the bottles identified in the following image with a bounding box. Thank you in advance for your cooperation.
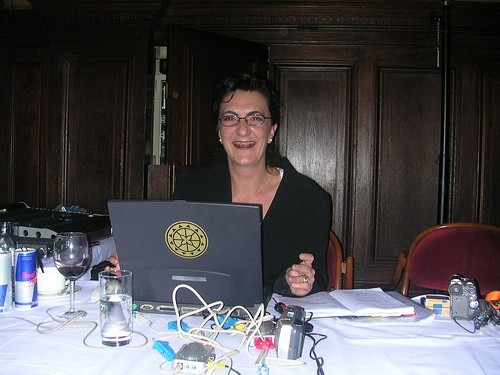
[0,220,17,303]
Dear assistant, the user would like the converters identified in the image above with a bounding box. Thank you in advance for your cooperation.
[173,341,216,375]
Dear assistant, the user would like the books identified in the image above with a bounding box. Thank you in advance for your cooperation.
[271,286,417,319]
[337,289,437,325]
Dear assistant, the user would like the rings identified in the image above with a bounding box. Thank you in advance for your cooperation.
[303,275,310,283]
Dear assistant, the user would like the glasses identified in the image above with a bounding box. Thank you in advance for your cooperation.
[217,113,273,127]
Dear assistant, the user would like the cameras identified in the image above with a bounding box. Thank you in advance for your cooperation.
[275,305,306,361]
[449,274,479,321]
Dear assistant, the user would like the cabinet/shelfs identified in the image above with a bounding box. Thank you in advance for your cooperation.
[0,1,500,291]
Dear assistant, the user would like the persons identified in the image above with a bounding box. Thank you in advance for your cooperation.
[103,69,334,299]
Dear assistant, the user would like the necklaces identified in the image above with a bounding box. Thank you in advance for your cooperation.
[230,167,268,206]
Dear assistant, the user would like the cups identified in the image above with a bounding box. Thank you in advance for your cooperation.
[98,270,134,346]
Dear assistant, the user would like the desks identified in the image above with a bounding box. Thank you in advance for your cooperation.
[0,232,500,375]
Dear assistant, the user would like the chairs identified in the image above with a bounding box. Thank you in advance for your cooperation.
[387,221,500,299]
[325,229,354,293]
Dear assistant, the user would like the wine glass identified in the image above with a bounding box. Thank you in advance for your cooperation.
[54,232,90,319]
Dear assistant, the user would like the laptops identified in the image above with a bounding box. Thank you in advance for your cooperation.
[108,199,273,319]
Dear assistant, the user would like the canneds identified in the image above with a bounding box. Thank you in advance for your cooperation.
[12,248,38,310]
[0,251,13,313]
